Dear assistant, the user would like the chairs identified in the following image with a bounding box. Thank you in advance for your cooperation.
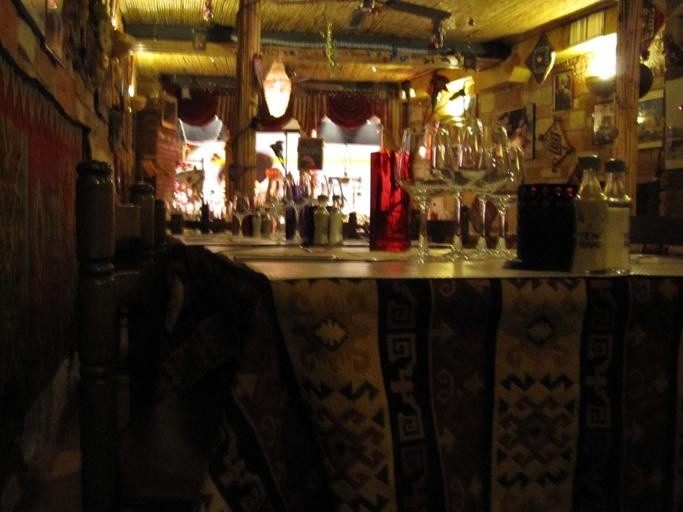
[75,160,199,512]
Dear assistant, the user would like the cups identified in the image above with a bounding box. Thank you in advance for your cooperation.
[368,150,416,253]
[516,183,578,272]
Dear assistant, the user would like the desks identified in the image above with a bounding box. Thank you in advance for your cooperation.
[167,231,683,511]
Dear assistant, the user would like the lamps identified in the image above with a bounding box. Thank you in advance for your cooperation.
[263,49,292,118]
[586,76,616,97]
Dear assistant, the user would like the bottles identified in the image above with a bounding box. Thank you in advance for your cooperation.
[571,153,611,276]
[328,195,344,247]
[223,177,297,241]
[604,157,635,273]
[311,195,330,246]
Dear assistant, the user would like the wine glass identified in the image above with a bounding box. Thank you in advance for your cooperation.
[465,122,514,264]
[396,126,456,264]
[431,114,495,264]
[490,145,525,263]
